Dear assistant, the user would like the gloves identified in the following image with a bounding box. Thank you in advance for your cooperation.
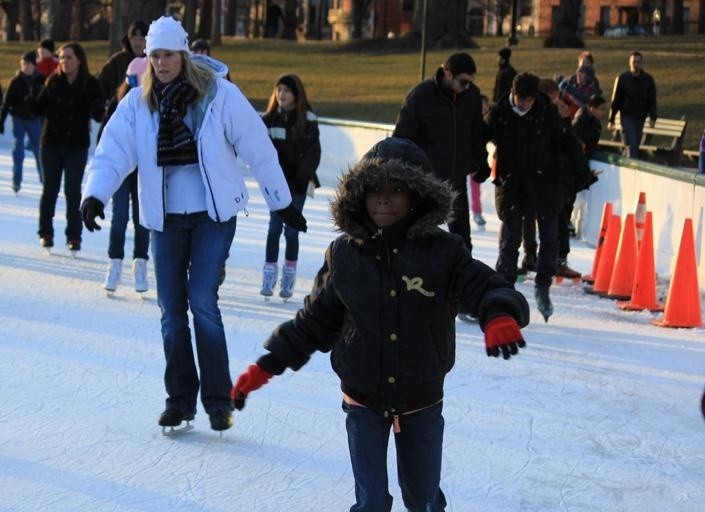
[81,195,105,231]
[482,316,526,361]
[230,364,273,410]
[276,202,308,233]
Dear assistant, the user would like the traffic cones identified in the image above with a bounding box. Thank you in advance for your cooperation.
[653,218,705,329]
[581,189,666,312]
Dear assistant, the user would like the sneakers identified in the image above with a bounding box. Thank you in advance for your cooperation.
[534,285,555,318]
[521,252,536,270]
[65,234,82,251]
[208,409,234,431]
[12,180,22,190]
[554,262,583,279]
[259,264,278,296]
[39,233,56,248]
[568,220,575,232]
[279,265,296,298]
[158,408,196,426]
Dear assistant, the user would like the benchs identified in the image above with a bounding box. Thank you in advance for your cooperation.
[597,109,687,166]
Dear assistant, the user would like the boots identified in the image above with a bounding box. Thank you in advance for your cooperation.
[131,256,150,292]
[103,256,123,291]
[473,212,486,225]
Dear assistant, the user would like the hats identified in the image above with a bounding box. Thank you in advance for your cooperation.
[40,38,62,52]
[144,11,193,57]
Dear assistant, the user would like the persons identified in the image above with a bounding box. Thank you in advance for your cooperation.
[79,17,308,431]
[229,137,530,512]
[391,53,492,314]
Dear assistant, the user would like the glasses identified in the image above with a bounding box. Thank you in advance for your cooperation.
[449,72,476,88]
